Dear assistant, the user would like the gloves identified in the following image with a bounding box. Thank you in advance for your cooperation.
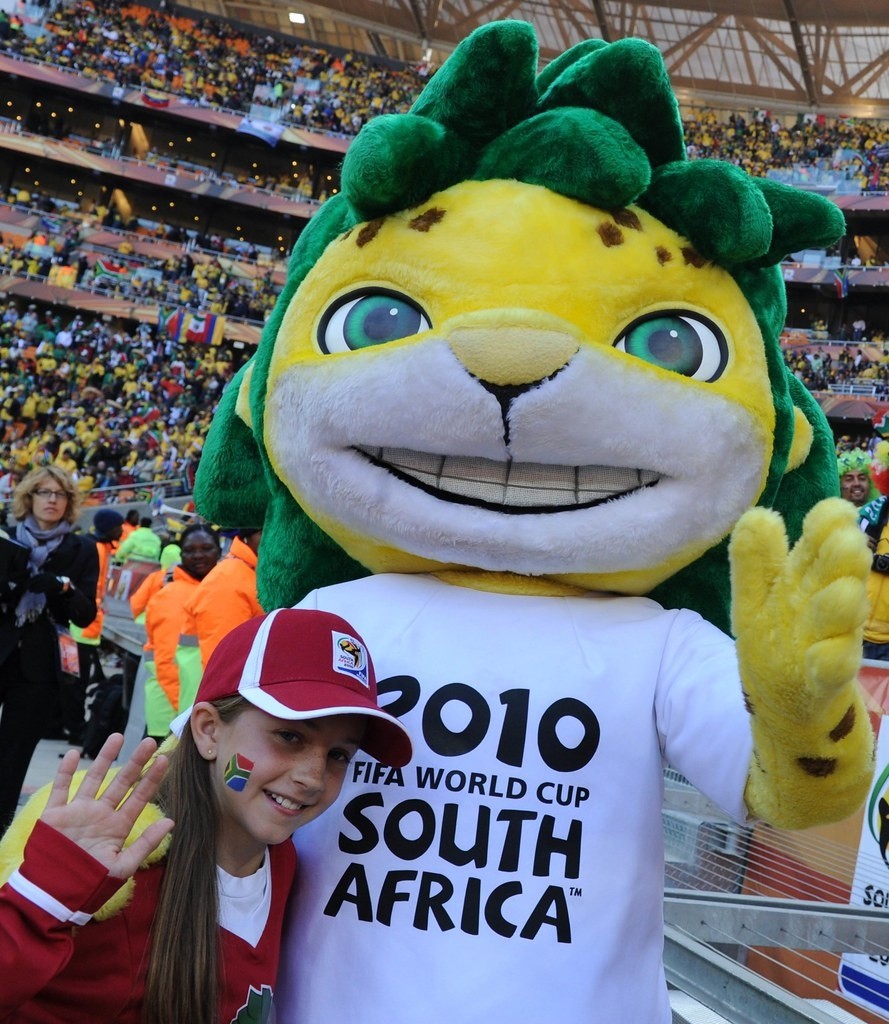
[28,574,63,594]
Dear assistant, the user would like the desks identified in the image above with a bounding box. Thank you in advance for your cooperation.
[102,615,198,767]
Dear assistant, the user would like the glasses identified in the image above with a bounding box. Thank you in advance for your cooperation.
[29,488,69,499]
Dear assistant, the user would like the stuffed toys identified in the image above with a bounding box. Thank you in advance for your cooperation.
[0,25,874,1023]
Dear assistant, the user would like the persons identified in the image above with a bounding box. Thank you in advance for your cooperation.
[1,2,888,838]
[0,609,412,1023]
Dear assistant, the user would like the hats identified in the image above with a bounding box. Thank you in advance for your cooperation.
[194,608,413,770]
[94,509,124,529]
